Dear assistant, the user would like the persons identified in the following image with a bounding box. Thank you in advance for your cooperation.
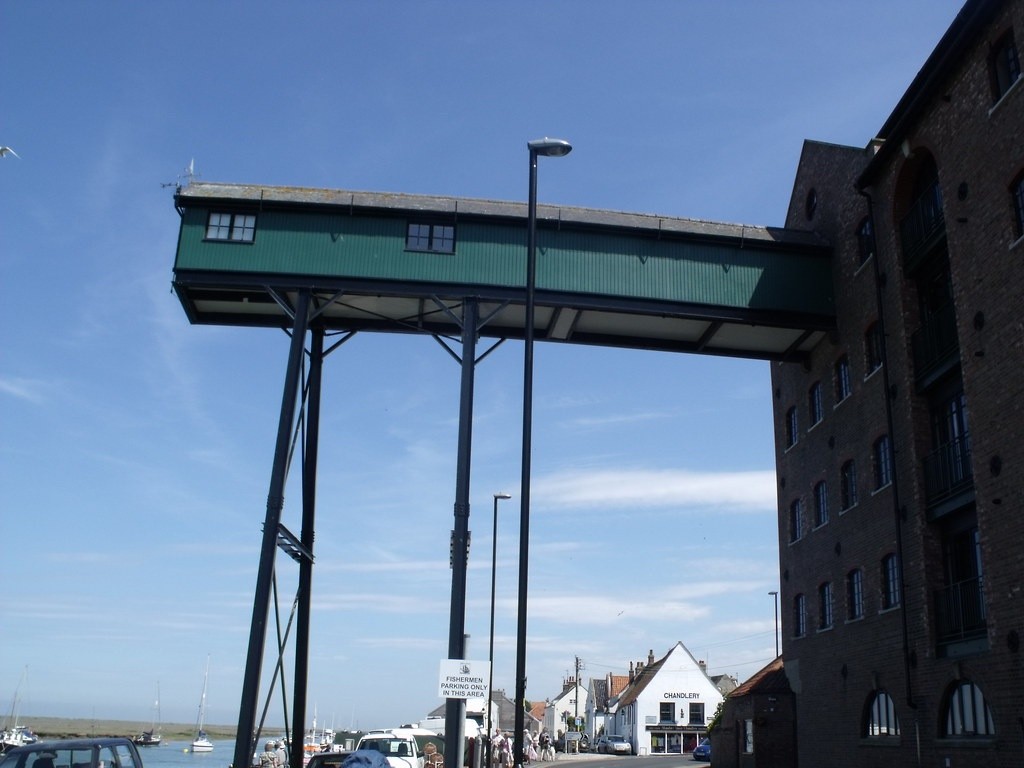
[274,741,288,768]
[522,729,533,765]
[499,731,514,768]
[259,742,277,768]
[532,730,540,755]
[490,727,505,768]
[538,726,551,762]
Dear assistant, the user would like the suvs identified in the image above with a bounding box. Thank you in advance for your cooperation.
[596,735,631,755]
[554,731,591,753]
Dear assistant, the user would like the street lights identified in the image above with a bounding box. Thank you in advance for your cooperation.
[768,591,780,658]
[512,134,573,766]
[486,492,511,768]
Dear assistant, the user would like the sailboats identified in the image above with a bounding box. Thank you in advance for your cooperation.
[189,653,213,753]
[282,700,361,767]
[135,680,162,745]
[0,665,44,751]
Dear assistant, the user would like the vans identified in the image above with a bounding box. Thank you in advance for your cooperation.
[355,733,426,768]
[0,737,143,768]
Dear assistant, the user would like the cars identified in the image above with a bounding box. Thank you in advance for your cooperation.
[693,736,712,761]
